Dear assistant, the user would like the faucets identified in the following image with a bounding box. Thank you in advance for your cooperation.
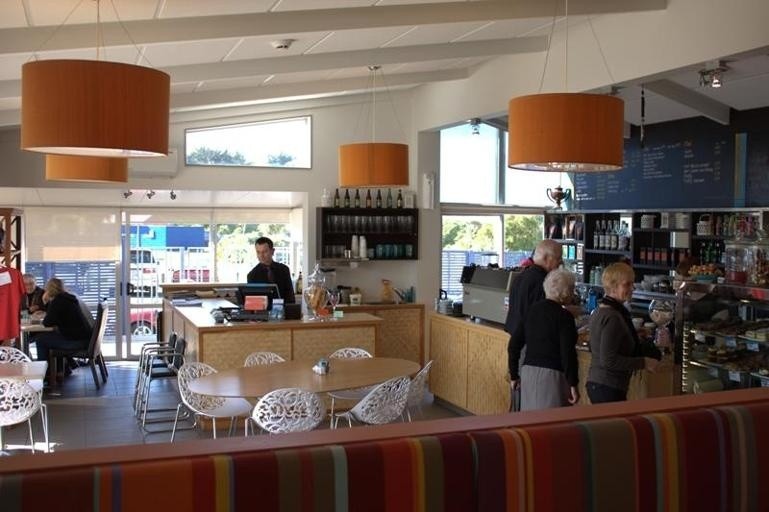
[392,287,405,300]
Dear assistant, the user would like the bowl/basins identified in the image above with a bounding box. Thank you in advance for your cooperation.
[690,274,715,282]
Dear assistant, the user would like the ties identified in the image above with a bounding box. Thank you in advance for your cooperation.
[266,266,273,282]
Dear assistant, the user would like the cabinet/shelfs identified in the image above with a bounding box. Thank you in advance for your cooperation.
[0,208,24,272]
[543,208,769,323]
[683,325,769,393]
[316,206,419,260]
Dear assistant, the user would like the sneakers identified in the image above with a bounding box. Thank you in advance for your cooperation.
[47,359,79,388]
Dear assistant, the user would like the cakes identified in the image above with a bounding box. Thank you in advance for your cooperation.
[691,315,769,376]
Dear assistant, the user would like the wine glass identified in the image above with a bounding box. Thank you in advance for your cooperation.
[328,292,339,320]
[647,297,674,347]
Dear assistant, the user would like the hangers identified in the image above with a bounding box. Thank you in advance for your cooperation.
[0,263,7,268]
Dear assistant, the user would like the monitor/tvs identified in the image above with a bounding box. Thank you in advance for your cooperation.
[626,290,675,321]
[238,283,279,310]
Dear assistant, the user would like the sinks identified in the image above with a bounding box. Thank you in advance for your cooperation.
[364,302,405,305]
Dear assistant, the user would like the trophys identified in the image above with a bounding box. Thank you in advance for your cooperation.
[546,185,571,210]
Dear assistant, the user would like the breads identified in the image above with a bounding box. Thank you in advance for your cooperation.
[311,286,323,309]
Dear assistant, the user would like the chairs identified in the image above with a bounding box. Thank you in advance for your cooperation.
[0,298,111,454]
[135,332,434,442]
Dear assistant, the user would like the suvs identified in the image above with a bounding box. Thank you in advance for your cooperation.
[106,246,160,296]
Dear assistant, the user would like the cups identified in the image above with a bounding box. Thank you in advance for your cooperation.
[638,246,685,267]
[21,309,44,329]
[324,215,412,259]
[215,316,224,324]
[640,215,653,228]
[642,322,655,337]
[631,317,644,330]
[337,285,362,305]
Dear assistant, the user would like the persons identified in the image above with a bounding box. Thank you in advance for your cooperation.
[248,237,297,304]
[23,274,49,366]
[505,241,565,411]
[37,278,98,378]
[507,269,579,411]
[585,261,669,404]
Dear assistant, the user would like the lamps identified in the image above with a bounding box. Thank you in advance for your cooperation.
[503,0,625,174]
[22,0,170,160]
[45,151,128,184]
[698,60,731,88]
[339,65,410,188]
[123,188,177,201]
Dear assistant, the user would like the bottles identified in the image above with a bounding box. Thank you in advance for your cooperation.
[697,242,724,265]
[319,361,327,374]
[584,266,604,313]
[380,279,394,304]
[723,229,769,288]
[291,272,302,295]
[737,300,752,322]
[715,213,758,238]
[633,269,678,295]
[304,263,331,322]
[695,214,713,236]
[593,218,630,250]
[321,187,415,209]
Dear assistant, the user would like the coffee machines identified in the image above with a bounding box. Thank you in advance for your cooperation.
[458,266,522,326]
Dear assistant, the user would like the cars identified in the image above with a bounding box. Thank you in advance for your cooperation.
[78,296,162,338]
[167,264,210,281]
